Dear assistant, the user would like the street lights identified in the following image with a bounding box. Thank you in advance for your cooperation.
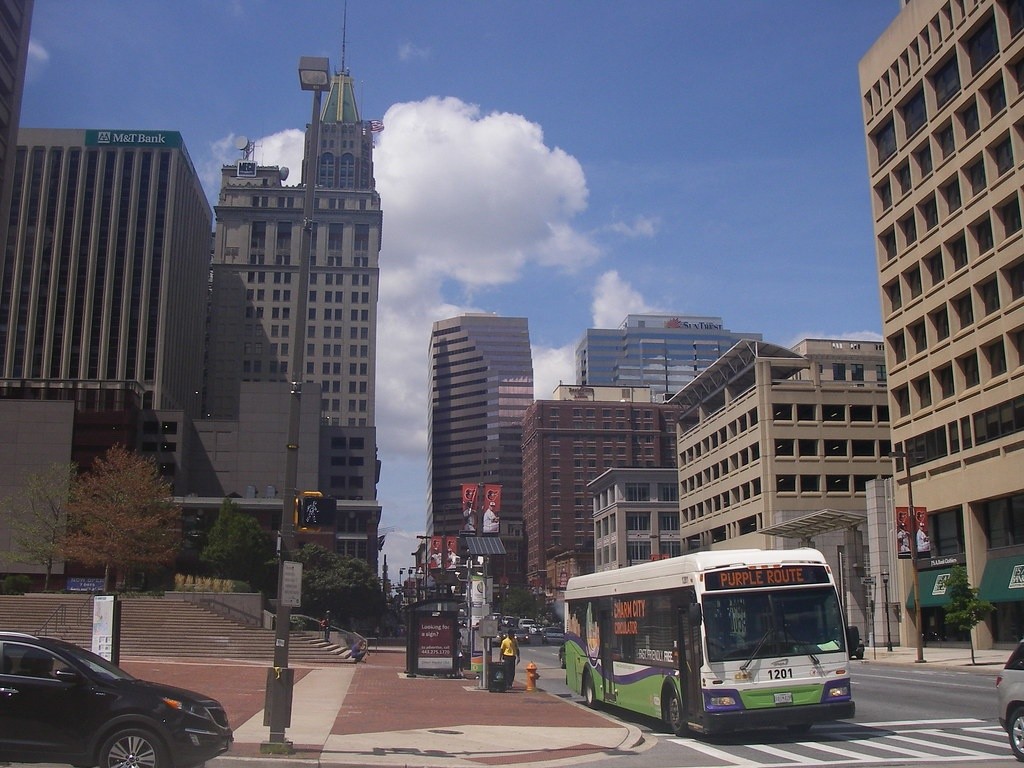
[417,536,431,600]
[888,450,928,664]
[263,56,317,743]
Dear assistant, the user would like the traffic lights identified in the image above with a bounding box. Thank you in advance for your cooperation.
[294,491,337,535]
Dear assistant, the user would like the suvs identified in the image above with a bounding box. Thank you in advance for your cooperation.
[0,631,235,768]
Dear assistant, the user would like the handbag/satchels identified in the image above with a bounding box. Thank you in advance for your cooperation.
[321,619,326,627]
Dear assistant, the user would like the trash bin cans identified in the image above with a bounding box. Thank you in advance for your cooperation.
[488,662,508,693]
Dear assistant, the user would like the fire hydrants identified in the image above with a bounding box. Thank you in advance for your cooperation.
[524,661,541,693]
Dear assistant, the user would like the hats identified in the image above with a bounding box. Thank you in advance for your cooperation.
[467,501,473,508]
[435,548,438,550]
[920,522,924,527]
[900,523,905,527]
[448,548,452,552]
[508,628,514,634]
[489,501,495,506]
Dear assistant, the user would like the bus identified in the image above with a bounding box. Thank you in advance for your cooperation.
[563,548,860,739]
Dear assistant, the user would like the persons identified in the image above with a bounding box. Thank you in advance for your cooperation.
[897,523,910,551]
[499,629,520,688]
[916,523,931,552]
[463,501,476,531]
[324,615,333,643]
[351,639,366,663]
[483,501,499,532]
[431,548,456,571]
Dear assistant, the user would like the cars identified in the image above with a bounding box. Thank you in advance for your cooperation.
[846,626,864,660]
[493,612,545,645]
[993,637,1024,763]
[542,626,563,646]
[559,643,566,670]
